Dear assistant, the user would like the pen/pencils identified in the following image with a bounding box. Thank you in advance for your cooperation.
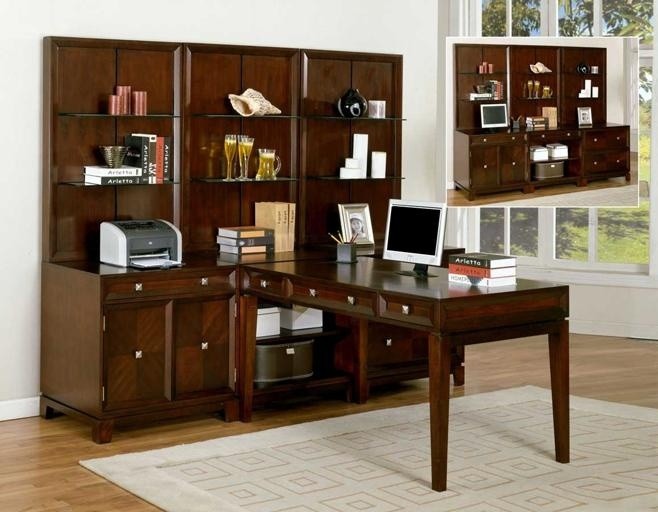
[349,233,355,244]
[328,233,341,244]
[352,233,359,243]
[337,230,344,244]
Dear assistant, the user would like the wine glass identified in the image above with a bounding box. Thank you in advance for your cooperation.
[222,134,255,182]
[527,80,540,98]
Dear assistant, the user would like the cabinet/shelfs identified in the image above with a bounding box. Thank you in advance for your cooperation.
[524,126,584,194]
[238,260,369,424]
[582,124,631,187]
[301,50,404,249]
[453,43,508,130]
[454,128,525,202]
[41,35,180,267]
[561,44,607,127]
[182,42,300,263]
[353,317,465,405]
[40,260,239,443]
[508,44,561,130]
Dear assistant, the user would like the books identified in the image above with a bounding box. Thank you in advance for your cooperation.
[525,115,546,128]
[447,251,519,280]
[82,164,142,185]
[215,225,275,256]
[541,106,558,128]
[123,131,171,181]
[448,274,518,288]
[254,200,296,254]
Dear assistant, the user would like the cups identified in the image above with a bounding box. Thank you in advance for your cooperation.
[513,121,520,129]
[257,149,281,181]
[542,85,553,98]
[335,242,357,263]
[368,100,386,119]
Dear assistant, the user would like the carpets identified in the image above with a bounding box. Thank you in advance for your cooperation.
[77,384,658,510]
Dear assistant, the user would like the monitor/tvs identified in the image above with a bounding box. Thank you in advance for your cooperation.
[382,199,448,279]
[480,103,508,133]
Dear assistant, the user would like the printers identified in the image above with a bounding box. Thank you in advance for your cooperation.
[100,218,186,271]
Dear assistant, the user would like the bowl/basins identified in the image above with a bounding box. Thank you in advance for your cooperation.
[99,146,130,168]
[476,85,486,93]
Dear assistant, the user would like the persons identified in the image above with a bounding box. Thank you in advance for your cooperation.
[349,213,367,239]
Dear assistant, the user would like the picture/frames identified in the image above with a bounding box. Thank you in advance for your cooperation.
[576,107,593,128]
[337,203,375,247]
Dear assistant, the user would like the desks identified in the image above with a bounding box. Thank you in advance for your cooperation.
[237,257,570,491]
